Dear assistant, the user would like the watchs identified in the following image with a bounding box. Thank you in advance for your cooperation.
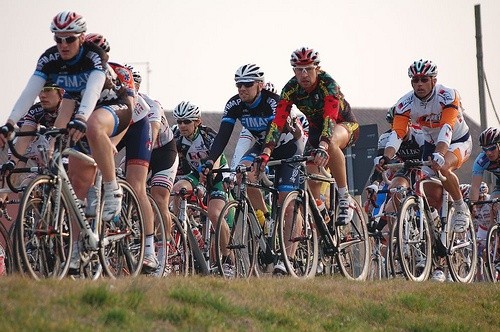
[316,144,329,152]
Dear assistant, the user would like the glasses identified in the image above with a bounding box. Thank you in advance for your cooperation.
[294,68,316,74]
[177,119,193,124]
[236,82,254,88]
[54,33,81,43]
[411,77,433,83]
[40,87,61,94]
[480,144,498,151]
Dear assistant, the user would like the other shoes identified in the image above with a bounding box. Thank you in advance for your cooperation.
[447,270,453,283]
[222,263,236,280]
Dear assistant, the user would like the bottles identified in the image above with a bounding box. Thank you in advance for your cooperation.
[192,226,204,247]
[86,185,99,217]
[255,209,265,228]
[315,199,331,223]
[430,207,440,226]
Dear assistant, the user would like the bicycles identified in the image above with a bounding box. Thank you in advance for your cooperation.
[0,128,500,283]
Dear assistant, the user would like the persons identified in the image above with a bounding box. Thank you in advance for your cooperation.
[170,47,360,274]
[0,11,180,277]
[364,59,500,282]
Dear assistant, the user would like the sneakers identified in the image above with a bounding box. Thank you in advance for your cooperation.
[431,270,446,283]
[452,202,470,233]
[70,243,82,269]
[273,260,295,274]
[102,184,123,221]
[316,255,323,274]
[143,252,159,270]
[336,197,358,225]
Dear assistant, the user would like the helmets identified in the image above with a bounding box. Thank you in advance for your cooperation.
[51,11,87,33]
[386,106,395,125]
[479,182,489,196]
[124,64,142,94]
[85,33,110,52]
[234,64,265,83]
[408,59,438,76]
[173,101,201,120]
[459,184,472,199]
[479,127,500,146]
[290,47,320,68]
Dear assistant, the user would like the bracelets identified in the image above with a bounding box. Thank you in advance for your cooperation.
[437,150,445,158]
